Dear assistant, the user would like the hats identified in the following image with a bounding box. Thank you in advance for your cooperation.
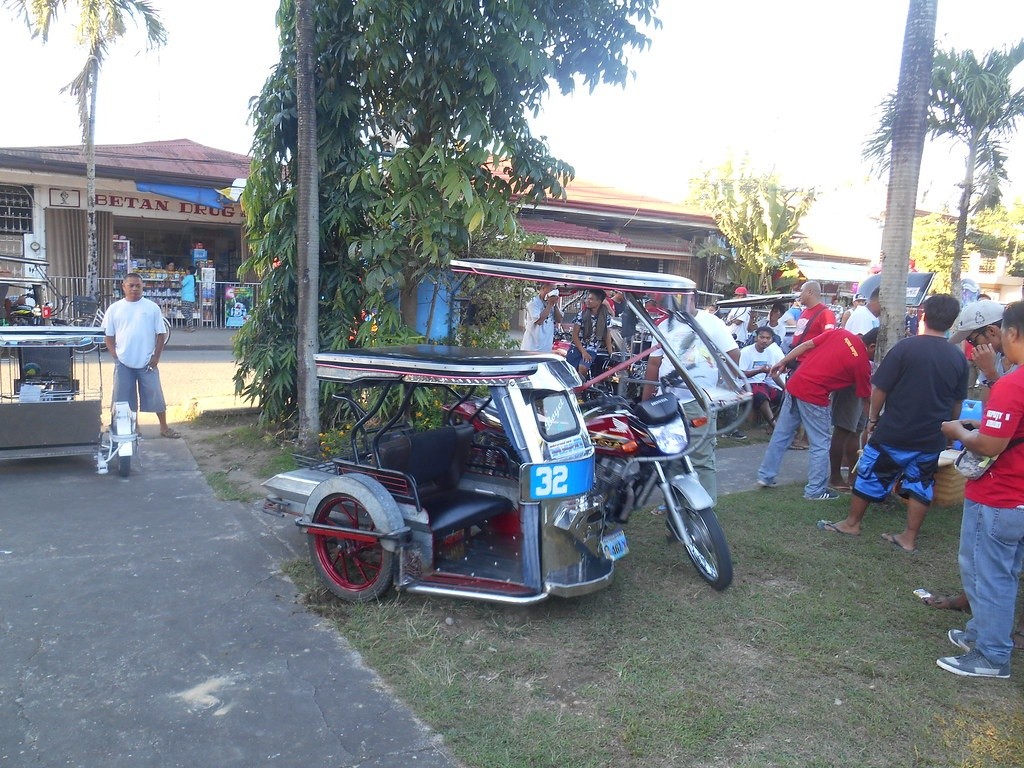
[951,300,1005,345]
[734,286,747,295]
[853,294,867,302]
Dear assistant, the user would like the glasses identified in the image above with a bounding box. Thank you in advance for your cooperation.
[968,330,986,347]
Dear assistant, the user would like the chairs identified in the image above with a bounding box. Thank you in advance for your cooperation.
[381,429,511,555]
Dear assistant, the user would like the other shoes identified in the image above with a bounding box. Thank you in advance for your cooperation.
[789,441,810,449]
[161,427,181,438]
[721,430,747,439]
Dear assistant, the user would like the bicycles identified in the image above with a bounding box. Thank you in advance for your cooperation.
[67,291,172,355]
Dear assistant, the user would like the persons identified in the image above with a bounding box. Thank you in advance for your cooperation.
[179,266,196,332]
[520,282,564,353]
[101,273,181,438]
[604,281,987,554]
[148,259,175,271]
[923,299,1024,678]
[565,289,613,376]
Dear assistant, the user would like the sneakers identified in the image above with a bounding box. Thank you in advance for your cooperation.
[806,490,839,500]
[937,647,1011,678]
[759,479,776,488]
[948,630,977,652]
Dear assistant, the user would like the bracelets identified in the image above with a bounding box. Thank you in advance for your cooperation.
[869,419,877,423]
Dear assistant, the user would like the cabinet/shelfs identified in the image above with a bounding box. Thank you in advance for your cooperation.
[138,278,204,320]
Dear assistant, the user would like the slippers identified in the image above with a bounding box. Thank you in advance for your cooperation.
[827,484,854,495]
[923,592,970,612]
[651,503,668,516]
[816,519,850,534]
[881,532,913,551]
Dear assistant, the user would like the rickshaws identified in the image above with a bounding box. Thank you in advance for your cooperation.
[551,291,801,410]
[258,257,754,609]
[0,254,143,479]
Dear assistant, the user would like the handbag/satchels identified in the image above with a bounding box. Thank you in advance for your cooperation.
[955,427,1001,479]
[786,350,796,369]
